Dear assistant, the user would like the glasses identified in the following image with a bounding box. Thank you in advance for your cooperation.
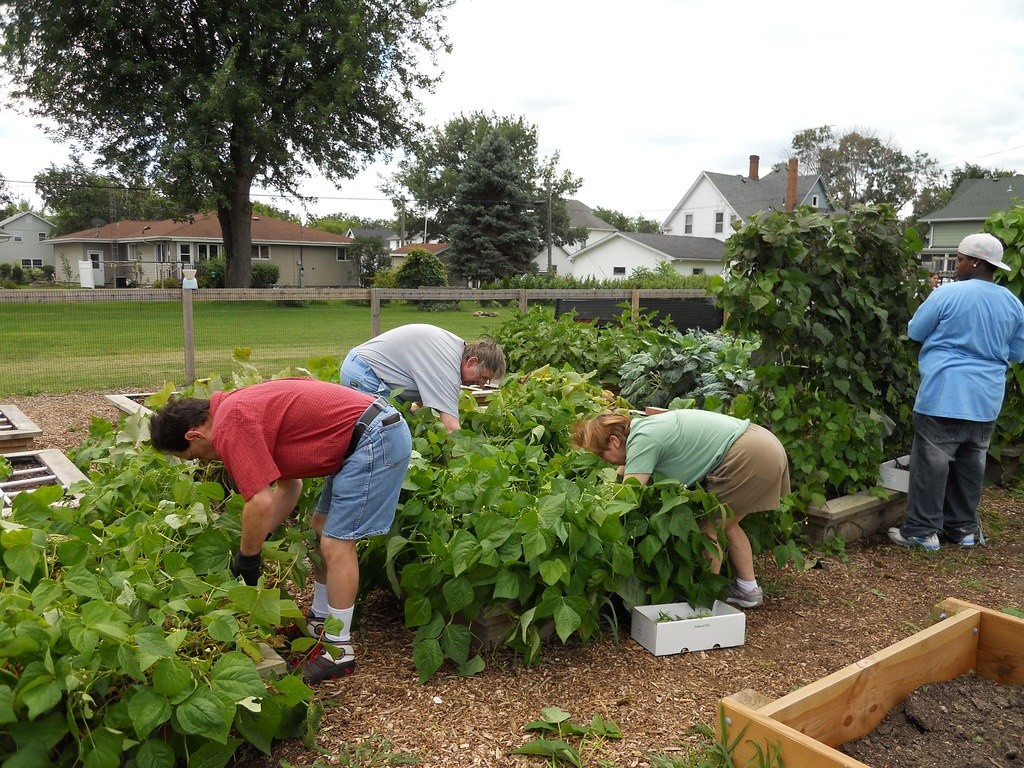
[186,442,206,468]
[477,362,487,388]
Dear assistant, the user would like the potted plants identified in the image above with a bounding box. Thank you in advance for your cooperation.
[0,206,1024,768]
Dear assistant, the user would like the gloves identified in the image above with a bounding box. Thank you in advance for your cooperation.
[232,533,270,590]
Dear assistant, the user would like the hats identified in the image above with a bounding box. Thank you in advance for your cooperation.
[958,231,1013,273]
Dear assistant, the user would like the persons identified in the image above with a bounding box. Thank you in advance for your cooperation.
[339,323,505,434]
[50,271,55,283]
[567,409,789,607]
[147,377,411,685]
[930,272,938,290]
[888,233,1023,551]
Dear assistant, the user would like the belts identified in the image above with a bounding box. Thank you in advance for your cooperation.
[340,395,395,466]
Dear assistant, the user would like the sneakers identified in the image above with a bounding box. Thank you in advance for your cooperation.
[722,577,764,609]
[941,524,976,549]
[888,526,941,553]
[268,608,334,655]
[281,638,356,684]
